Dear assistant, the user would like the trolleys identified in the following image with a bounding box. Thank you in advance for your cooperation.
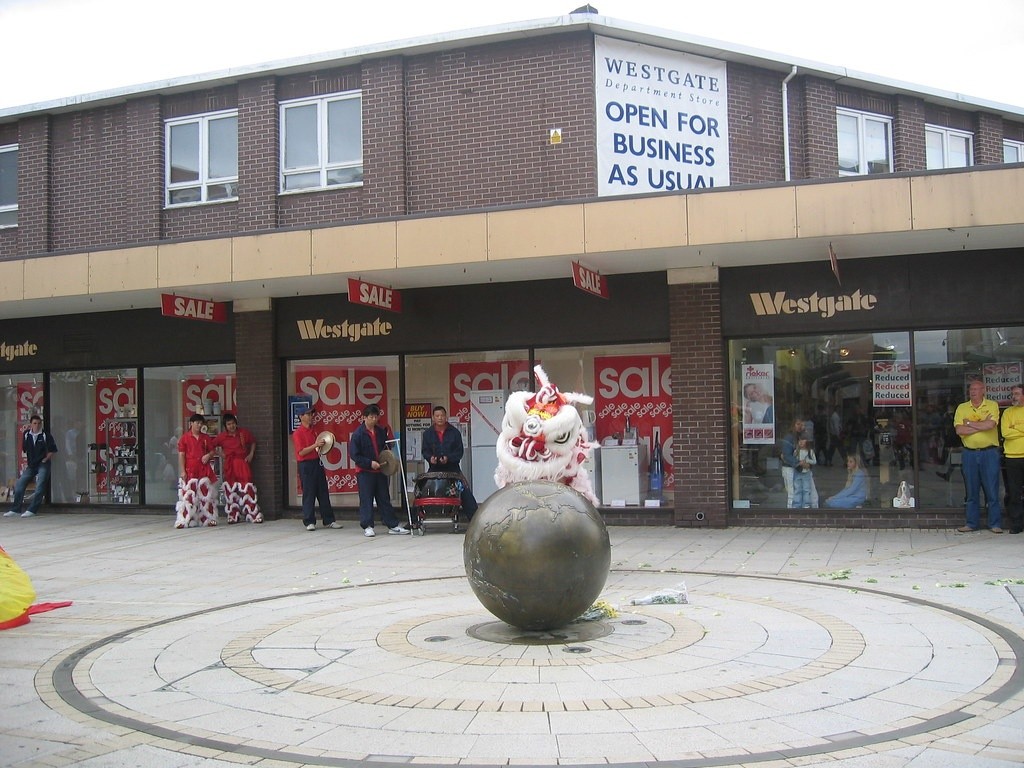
[417,506,459,536]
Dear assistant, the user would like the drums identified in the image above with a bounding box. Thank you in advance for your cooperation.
[413,472,463,519]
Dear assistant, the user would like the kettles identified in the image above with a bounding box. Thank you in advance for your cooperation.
[582,410,596,425]
[586,423,593,441]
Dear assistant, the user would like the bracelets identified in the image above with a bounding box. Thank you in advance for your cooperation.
[966,421,970,424]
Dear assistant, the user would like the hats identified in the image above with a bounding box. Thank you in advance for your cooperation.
[298,407,315,417]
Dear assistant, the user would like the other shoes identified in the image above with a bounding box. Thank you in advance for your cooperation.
[1010,527,1023,534]
[990,527,1003,532]
[3,511,22,517]
[958,526,974,531]
[403,523,411,530]
[22,511,34,518]
[935,471,950,482]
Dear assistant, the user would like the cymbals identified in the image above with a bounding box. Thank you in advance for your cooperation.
[315,431,336,455]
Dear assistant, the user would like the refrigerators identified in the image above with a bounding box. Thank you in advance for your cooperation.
[469,390,509,504]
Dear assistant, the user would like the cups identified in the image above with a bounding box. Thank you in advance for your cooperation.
[195,402,221,416]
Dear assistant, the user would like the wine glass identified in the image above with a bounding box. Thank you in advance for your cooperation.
[120,450,130,458]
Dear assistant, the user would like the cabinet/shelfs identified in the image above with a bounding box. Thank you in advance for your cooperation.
[86,444,108,500]
[105,418,140,503]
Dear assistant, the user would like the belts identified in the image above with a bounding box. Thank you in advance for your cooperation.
[965,445,997,450]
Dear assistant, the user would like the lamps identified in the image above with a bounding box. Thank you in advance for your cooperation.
[995,330,1007,347]
[179,370,191,382]
[7,378,17,389]
[32,377,41,389]
[115,374,125,386]
[820,339,832,355]
[88,374,95,386]
[205,368,215,381]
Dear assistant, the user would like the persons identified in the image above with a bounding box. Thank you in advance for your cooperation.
[213,414,264,523]
[1000,384,1024,534]
[823,454,871,510]
[349,405,411,537]
[51,415,91,504]
[801,404,878,467]
[781,420,820,509]
[175,415,218,527]
[292,407,344,531]
[952,380,1003,533]
[891,401,961,481]
[403,405,478,529]
[3,415,58,518]
[743,383,773,423]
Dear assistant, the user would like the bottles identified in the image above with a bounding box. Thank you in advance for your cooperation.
[115,444,130,456]
[74,491,90,504]
[2,486,14,497]
[123,424,136,437]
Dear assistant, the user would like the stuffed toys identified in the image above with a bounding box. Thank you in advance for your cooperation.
[494,363,602,508]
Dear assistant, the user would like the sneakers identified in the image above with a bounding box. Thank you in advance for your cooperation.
[389,526,410,535]
[325,522,343,528]
[307,524,315,531]
[363,527,375,537]
